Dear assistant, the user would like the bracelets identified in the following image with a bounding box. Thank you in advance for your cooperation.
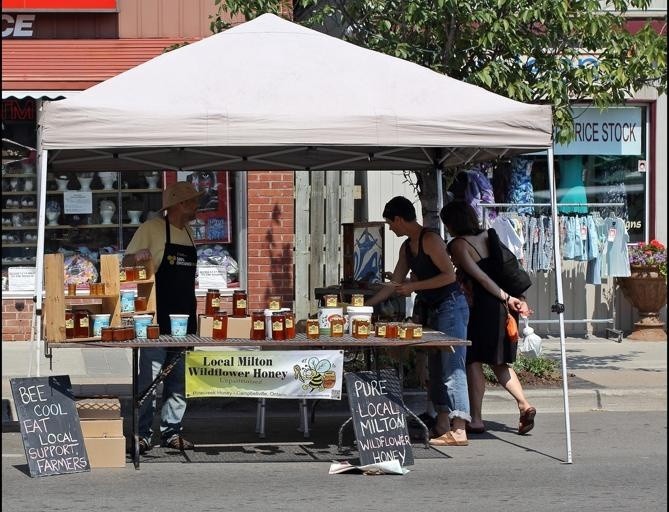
[503,294,510,304]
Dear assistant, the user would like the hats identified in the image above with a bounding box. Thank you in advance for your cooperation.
[156,181,207,214]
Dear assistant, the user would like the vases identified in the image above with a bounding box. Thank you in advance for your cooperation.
[615,265,668,343]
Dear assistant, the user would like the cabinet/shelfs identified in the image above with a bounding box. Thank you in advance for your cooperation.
[0,170,162,343]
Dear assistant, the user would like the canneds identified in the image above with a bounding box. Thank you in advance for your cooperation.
[65,308,90,339]
[119,264,147,282]
[331,318,344,337]
[101,325,135,341]
[205,289,220,317]
[134,297,147,311]
[351,294,365,307]
[306,319,320,339]
[323,371,336,389]
[352,317,423,339]
[147,324,160,339]
[325,295,338,308]
[233,289,246,318]
[252,296,296,340]
[213,311,228,340]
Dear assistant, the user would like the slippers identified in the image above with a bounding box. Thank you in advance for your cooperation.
[519,407,536,435]
[428,431,468,446]
[465,423,485,434]
[409,427,440,440]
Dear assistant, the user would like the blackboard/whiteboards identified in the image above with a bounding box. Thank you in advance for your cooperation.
[9,375,91,478]
[344,368,414,465]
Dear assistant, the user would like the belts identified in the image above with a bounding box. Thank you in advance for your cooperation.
[442,291,462,303]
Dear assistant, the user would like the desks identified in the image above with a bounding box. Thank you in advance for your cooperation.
[43,323,474,471]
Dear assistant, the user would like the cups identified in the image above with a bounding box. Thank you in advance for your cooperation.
[168,314,189,338]
[133,315,153,338]
[96,283,105,295]
[25,181,33,192]
[89,282,97,295]
[67,282,76,296]
[90,314,112,338]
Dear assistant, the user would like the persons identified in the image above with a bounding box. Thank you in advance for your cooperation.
[447,238,472,308]
[46,172,160,225]
[441,201,536,435]
[558,155,588,214]
[506,158,535,215]
[365,196,472,446]
[122,181,206,453]
[411,228,440,428]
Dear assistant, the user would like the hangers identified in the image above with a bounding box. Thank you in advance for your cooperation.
[500,206,618,224]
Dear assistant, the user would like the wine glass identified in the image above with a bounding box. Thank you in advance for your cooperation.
[10,178,18,191]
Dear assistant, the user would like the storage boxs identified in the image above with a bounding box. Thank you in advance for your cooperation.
[197,313,253,339]
[77,395,126,469]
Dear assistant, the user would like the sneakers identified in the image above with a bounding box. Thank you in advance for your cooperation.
[168,437,194,449]
[139,439,148,453]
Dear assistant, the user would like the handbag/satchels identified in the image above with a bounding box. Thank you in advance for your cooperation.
[507,313,518,345]
[487,228,532,297]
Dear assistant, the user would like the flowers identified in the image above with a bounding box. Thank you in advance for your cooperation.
[626,239,668,279]
